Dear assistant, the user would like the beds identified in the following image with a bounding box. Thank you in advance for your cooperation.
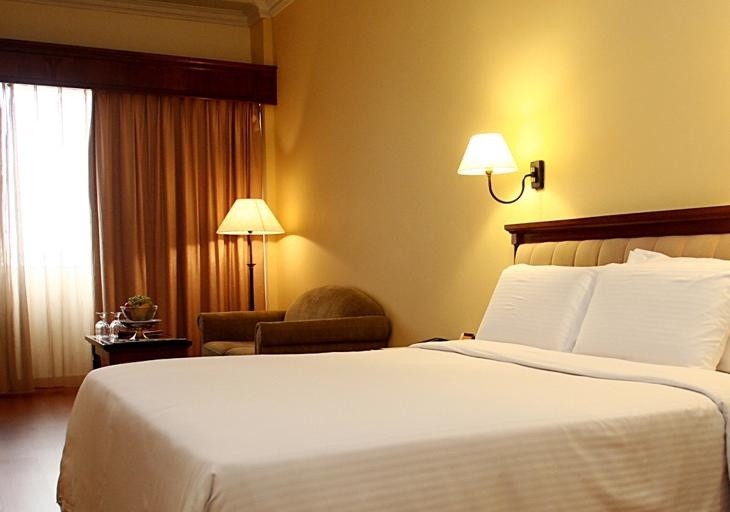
[79,205,730,511]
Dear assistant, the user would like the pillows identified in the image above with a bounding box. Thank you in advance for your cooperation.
[476,256,729,377]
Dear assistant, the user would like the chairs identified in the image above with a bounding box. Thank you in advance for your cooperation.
[197,284,391,356]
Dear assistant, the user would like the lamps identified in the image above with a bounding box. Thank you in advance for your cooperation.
[457,133,544,206]
[216,197,286,310]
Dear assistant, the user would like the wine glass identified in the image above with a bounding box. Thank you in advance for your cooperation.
[109,311,129,342]
[95,311,111,340]
